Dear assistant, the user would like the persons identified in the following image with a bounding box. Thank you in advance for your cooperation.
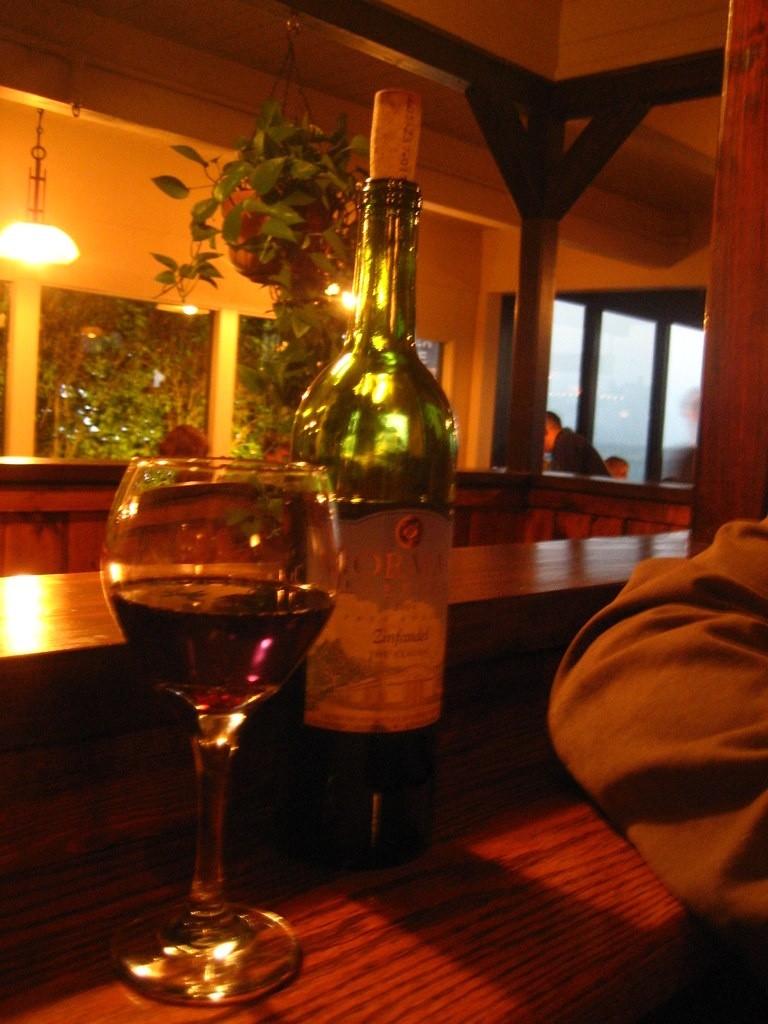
[546,513,768,951]
[604,457,629,479]
[542,412,611,477]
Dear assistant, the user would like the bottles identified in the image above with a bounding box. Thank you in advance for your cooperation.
[288,177,460,873]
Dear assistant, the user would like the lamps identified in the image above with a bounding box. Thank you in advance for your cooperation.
[0,108,80,265]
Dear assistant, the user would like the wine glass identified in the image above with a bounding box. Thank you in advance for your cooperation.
[100,454,338,1009]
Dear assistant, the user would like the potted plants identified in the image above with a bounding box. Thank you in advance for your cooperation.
[150,98,369,403]
